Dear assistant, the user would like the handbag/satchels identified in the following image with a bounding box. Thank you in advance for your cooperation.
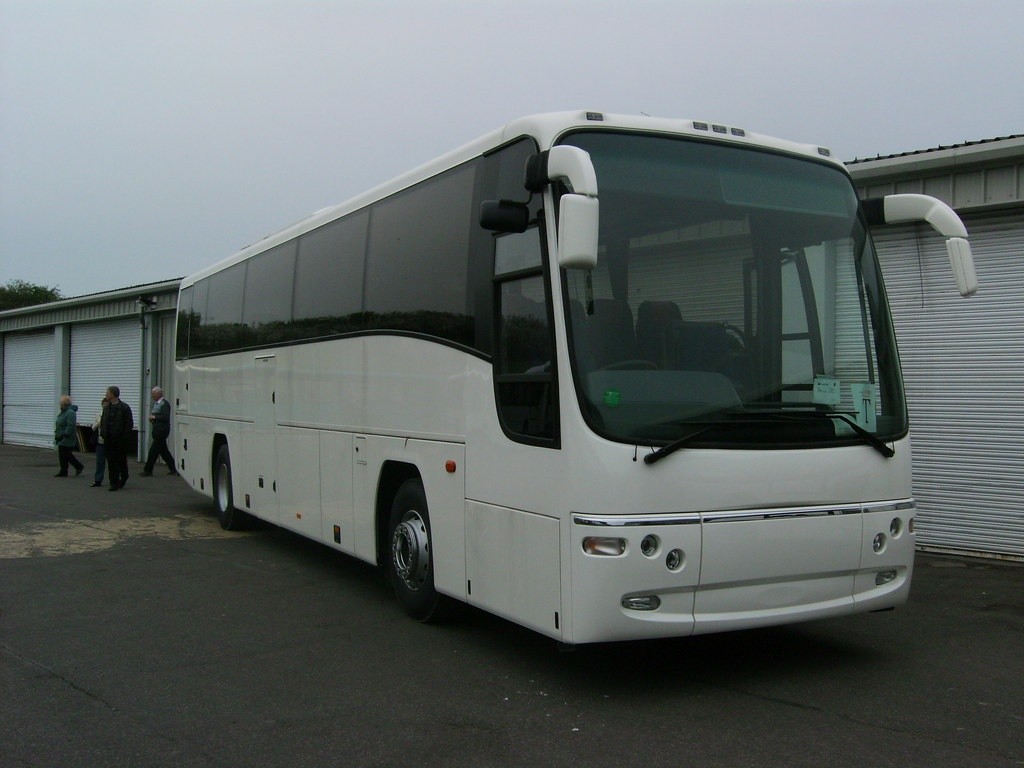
[55,432,64,441]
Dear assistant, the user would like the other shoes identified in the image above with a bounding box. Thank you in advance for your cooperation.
[167,471,178,476]
[118,479,125,488]
[108,486,118,491]
[76,465,84,476]
[138,471,153,477]
[54,472,68,477]
[90,481,102,487]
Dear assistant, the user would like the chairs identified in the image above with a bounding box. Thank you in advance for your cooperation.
[588,298,634,351]
[636,300,681,347]
[541,299,588,339]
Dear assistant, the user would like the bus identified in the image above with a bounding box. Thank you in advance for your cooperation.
[169,107,980,650]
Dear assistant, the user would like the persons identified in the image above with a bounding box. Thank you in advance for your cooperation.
[89,397,113,486]
[139,387,179,476]
[53,396,85,477]
[101,386,134,492]
